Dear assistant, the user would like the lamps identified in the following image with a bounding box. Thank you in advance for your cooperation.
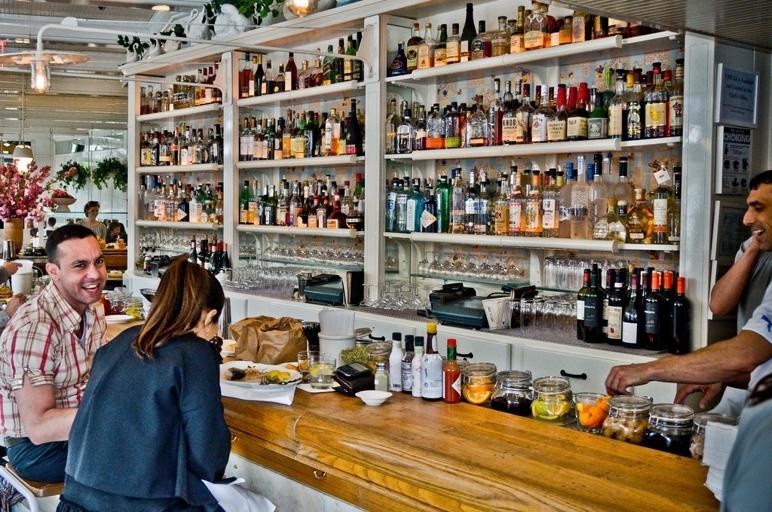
[12,74,33,159]
[0,17,380,95]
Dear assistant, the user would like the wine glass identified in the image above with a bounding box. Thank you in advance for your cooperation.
[137,227,365,266]
[360,249,520,312]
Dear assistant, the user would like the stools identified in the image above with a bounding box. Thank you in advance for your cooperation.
[0,464,65,512]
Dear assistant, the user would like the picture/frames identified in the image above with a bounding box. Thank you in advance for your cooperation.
[714,62,760,129]
[715,126,754,195]
[710,200,752,261]
[708,260,738,321]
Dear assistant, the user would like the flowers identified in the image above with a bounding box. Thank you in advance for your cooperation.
[0,160,58,225]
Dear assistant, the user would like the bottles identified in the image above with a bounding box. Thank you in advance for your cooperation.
[140,173,225,225]
[140,116,222,170]
[383,148,681,247]
[236,171,365,232]
[355,322,736,462]
[236,97,367,158]
[379,0,652,78]
[232,29,367,98]
[144,255,152,276]
[383,57,682,156]
[190,234,230,274]
[101,291,111,315]
[577,264,692,354]
[142,61,226,115]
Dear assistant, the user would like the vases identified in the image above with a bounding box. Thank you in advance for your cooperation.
[4,218,23,253]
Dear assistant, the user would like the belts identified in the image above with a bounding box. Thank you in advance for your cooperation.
[5,437,25,448]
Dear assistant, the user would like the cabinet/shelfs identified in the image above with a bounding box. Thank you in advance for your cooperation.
[120,1,684,403]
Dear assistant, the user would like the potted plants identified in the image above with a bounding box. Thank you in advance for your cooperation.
[52,160,89,191]
[91,157,128,193]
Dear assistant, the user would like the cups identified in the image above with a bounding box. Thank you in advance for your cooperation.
[10,259,33,296]
[309,355,337,389]
[3,239,16,260]
[519,256,633,338]
[225,264,335,292]
[297,350,326,384]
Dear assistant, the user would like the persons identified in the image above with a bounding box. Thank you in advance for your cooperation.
[0,292,28,329]
[56,256,232,512]
[23,201,127,248]
[0,261,22,284]
[710,234,772,336]
[0,224,112,483]
[606,170,772,411]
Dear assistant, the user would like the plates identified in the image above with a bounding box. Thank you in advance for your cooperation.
[220,339,236,357]
[220,361,303,389]
[106,315,133,324]
